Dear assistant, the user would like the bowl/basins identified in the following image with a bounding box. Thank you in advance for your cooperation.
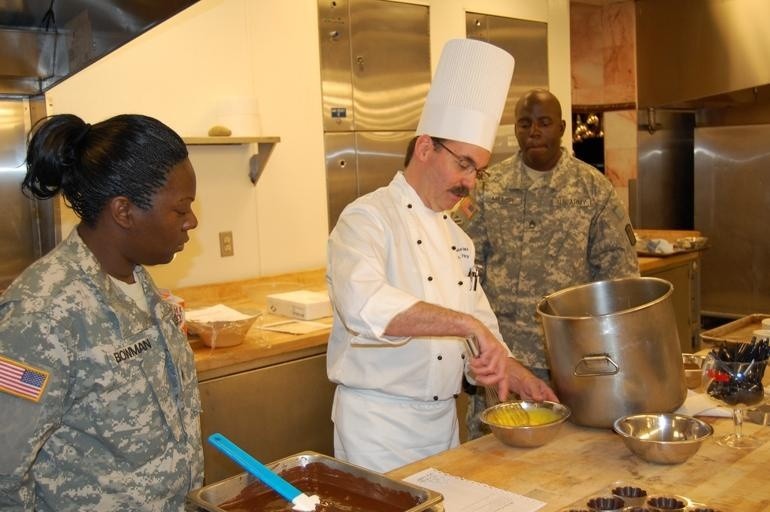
[678,235,708,249]
[479,399,572,450]
[181,304,263,349]
[680,352,706,389]
[614,412,715,466]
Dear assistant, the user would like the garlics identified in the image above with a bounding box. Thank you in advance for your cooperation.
[576,116,598,138]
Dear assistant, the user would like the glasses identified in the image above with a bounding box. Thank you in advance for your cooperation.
[430,138,490,181]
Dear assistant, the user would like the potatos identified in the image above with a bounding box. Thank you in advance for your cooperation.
[208,126,231,137]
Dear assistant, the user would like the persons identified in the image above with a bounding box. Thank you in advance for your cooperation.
[0,112,204,511]
[324,133,562,475]
[451,91,641,442]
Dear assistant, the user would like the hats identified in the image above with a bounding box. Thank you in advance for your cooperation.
[414,38,516,154]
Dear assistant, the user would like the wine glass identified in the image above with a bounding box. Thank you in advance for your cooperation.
[699,356,768,454]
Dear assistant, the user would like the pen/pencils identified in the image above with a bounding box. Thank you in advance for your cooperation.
[468,268,478,291]
